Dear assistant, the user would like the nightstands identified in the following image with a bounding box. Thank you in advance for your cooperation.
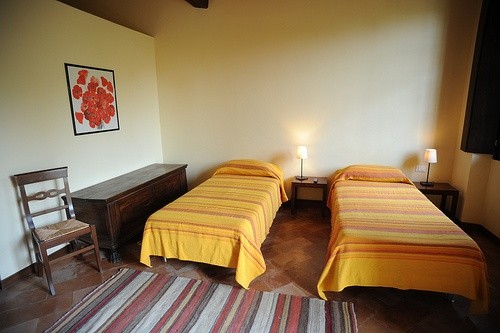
[291,177,327,216]
[414,182,460,220]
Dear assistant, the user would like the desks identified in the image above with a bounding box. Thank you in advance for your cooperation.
[67,165,188,265]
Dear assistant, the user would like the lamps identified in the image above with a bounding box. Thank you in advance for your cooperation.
[420,149,439,187]
[295,146,308,180]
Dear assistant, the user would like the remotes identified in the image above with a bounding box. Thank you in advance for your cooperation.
[314,179,318,183]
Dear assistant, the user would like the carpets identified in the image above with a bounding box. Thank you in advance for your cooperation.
[47,273,364,333]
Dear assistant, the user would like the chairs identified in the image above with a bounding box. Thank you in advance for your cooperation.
[16,165,105,299]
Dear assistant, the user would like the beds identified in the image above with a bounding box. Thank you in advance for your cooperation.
[318,165,491,315]
[146,161,291,292]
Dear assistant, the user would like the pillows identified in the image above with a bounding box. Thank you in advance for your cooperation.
[333,166,404,182]
[216,160,278,178]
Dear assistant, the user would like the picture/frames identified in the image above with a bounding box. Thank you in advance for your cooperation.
[64,62,121,136]
